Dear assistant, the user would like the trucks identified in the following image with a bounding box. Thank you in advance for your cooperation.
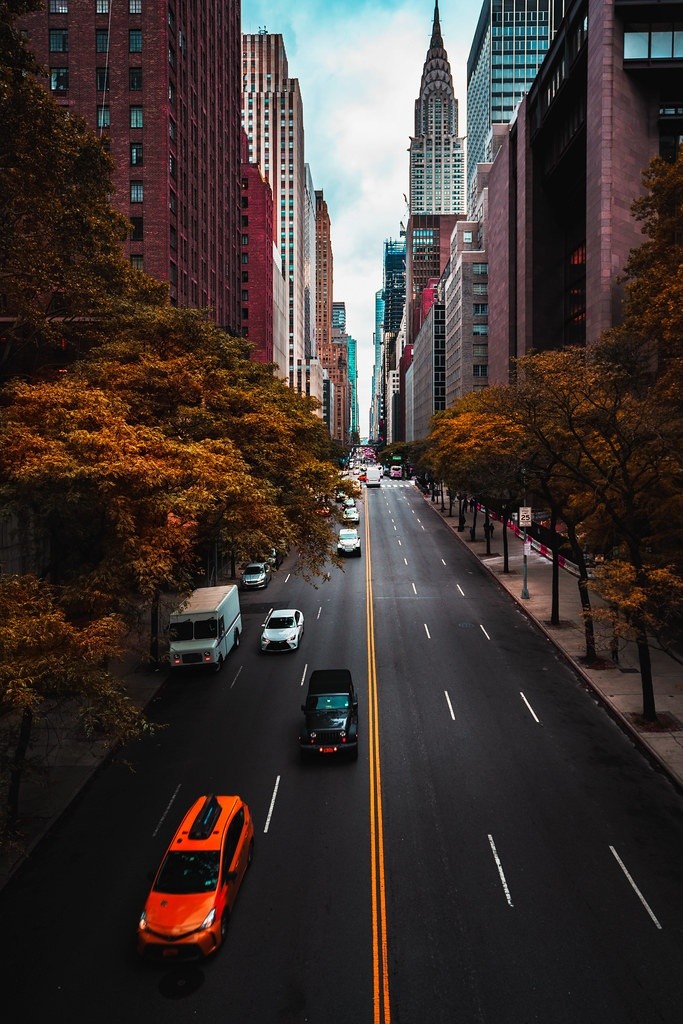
[163,585,243,675]
[365,467,381,488]
[376,466,383,478]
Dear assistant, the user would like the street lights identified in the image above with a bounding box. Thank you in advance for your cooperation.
[521,468,528,599]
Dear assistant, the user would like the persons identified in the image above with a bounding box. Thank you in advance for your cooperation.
[490,523,494,537]
[469,498,474,512]
[463,498,468,513]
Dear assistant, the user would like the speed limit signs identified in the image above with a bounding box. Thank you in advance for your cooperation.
[520,507,531,527]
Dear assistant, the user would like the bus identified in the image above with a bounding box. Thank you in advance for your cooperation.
[390,466,403,479]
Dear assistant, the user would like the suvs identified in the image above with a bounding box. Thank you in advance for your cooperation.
[300,669,359,763]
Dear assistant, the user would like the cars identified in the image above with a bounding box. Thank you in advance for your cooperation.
[343,459,366,482]
[138,795,254,962]
[342,498,356,511]
[261,609,304,653]
[342,507,360,525]
[335,491,349,503]
[240,562,272,591]
[336,529,361,557]
[256,547,284,570]
